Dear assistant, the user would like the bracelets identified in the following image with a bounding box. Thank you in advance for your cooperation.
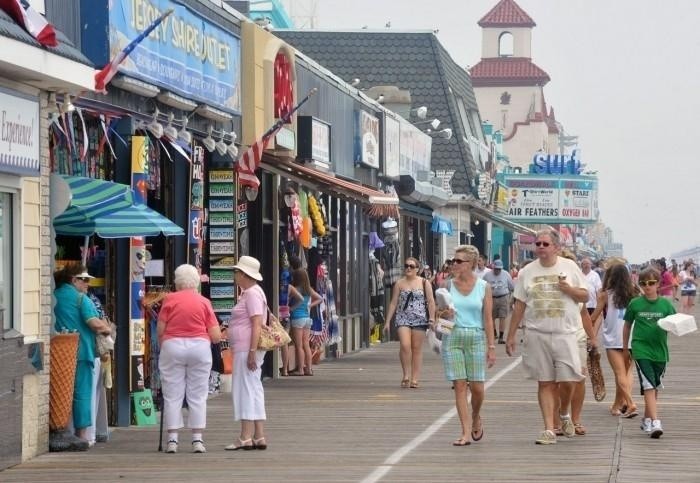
[249,349,255,352]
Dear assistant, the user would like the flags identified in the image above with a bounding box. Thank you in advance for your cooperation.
[94,17,164,90]
[234,111,291,190]
[0,0,59,47]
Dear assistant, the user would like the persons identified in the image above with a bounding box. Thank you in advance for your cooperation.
[591,260,644,420]
[429,253,699,346]
[619,266,676,439]
[51,260,114,448]
[501,225,591,447]
[279,254,324,376]
[221,253,273,452]
[154,262,224,456]
[435,244,495,447]
[381,256,437,390]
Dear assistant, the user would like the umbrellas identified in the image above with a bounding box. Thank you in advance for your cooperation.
[55,199,185,268]
[51,177,132,229]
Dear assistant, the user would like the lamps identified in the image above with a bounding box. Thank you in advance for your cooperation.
[395,106,427,119]
[413,119,440,129]
[156,91,198,112]
[202,124,239,158]
[149,106,191,145]
[45,86,76,113]
[111,75,161,97]
[425,128,452,140]
[195,104,233,123]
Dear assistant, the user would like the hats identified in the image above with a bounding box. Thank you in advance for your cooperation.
[492,260,505,270]
[231,255,263,281]
[72,272,96,279]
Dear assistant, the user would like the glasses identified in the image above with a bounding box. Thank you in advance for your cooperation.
[452,258,469,264]
[536,240,556,246]
[405,263,416,269]
[640,279,658,287]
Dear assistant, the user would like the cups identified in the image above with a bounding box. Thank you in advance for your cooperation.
[138,381,144,390]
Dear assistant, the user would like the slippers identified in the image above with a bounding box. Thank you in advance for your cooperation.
[472,414,484,441]
[453,438,471,448]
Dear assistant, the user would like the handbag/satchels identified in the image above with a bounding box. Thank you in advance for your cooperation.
[255,312,291,352]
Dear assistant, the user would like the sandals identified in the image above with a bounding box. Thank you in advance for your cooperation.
[224,437,253,452]
[609,405,639,418]
[254,438,269,450]
[574,421,587,435]
[278,364,315,377]
[397,378,419,388]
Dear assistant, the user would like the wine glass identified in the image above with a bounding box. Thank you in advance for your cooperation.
[137,358,143,379]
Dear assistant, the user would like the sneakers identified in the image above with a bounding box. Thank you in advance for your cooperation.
[560,412,576,439]
[535,430,557,444]
[191,440,207,452]
[164,440,178,454]
[498,339,506,344]
[641,417,652,434]
[650,419,664,440]
